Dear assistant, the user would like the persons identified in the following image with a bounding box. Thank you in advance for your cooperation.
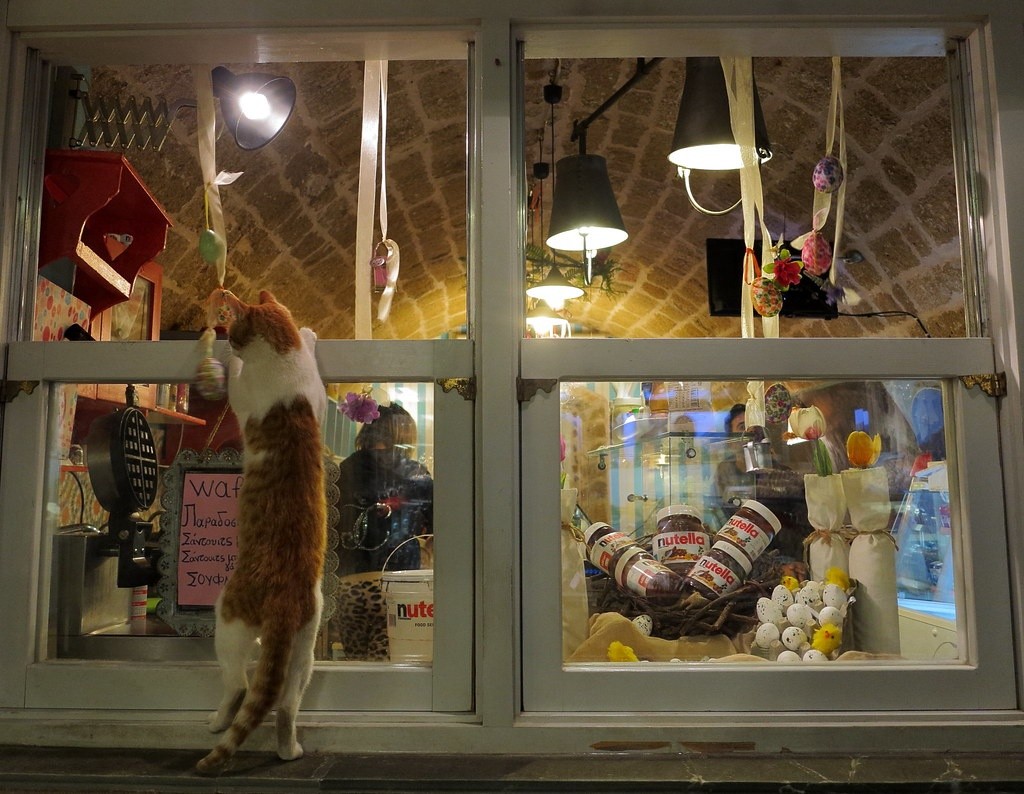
[335,403,434,661]
[715,403,805,559]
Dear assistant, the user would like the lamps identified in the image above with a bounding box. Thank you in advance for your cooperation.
[54,63,298,153]
[526,56,774,336]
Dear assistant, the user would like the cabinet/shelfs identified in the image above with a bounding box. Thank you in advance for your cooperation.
[318,396,358,459]
[36,144,208,443]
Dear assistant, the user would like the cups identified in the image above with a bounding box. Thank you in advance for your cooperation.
[743,441,772,475]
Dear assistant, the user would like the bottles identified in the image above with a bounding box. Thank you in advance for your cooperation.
[579,498,783,601]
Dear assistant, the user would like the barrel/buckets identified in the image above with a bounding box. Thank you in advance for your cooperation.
[381,533,433,664]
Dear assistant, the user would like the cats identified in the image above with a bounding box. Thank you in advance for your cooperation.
[196,290,331,774]
